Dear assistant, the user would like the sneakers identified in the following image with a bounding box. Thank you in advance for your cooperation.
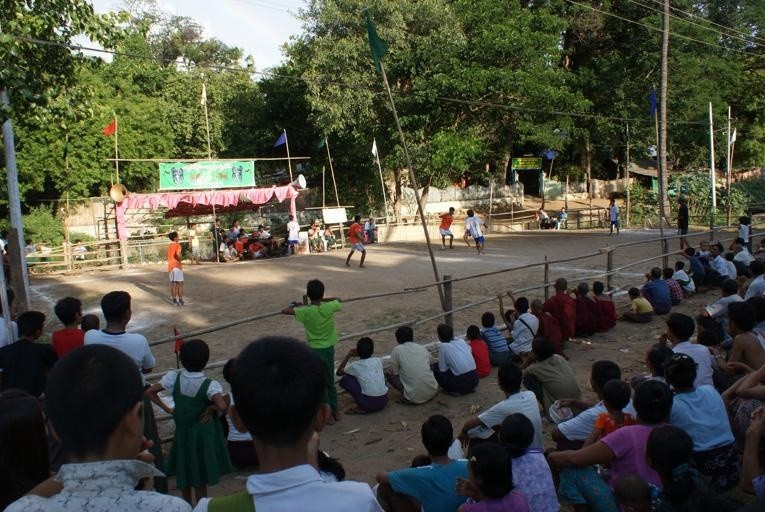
[172,298,185,305]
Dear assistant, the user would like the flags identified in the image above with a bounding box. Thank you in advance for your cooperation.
[273,132,285,148]
[317,138,325,148]
[649,90,657,116]
[729,129,737,144]
[200,87,207,108]
[371,141,377,156]
[104,118,116,136]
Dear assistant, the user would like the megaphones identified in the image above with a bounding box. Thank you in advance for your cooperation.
[109,183,128,202]
[287,173,308,190]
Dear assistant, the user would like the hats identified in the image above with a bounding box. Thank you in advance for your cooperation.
[549,400,575,424]
[467,422,495,440]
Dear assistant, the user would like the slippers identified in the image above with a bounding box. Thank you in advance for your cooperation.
[344,407,367,415]
